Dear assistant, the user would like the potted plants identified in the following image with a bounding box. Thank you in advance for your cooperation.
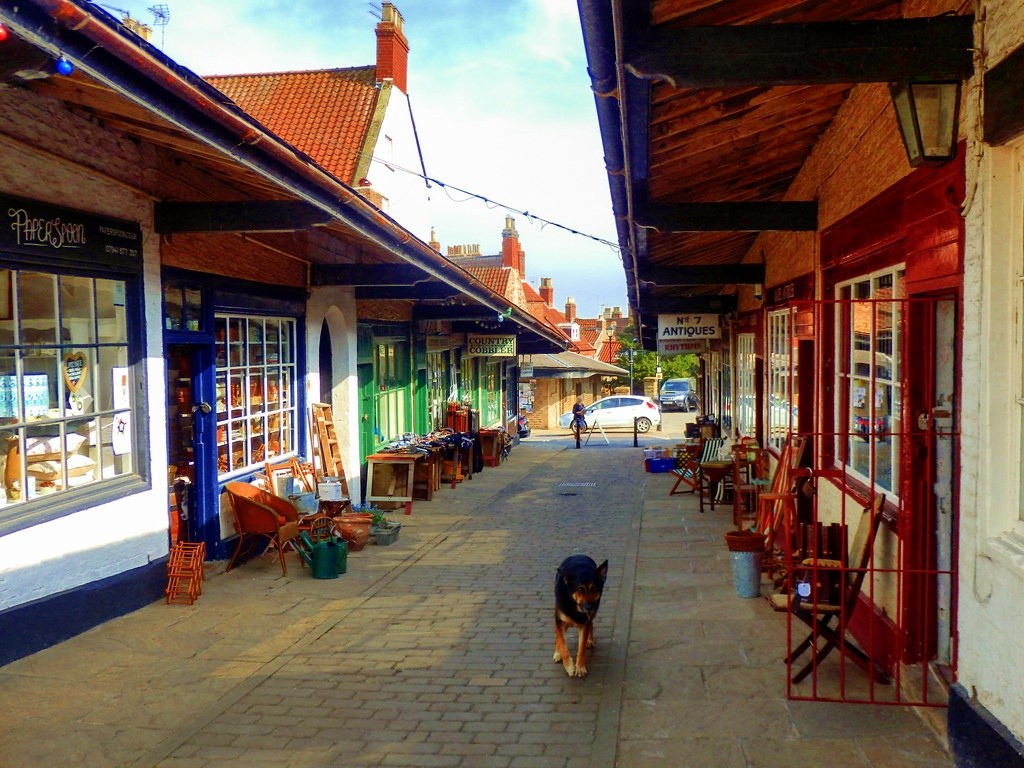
[359,507,383,536]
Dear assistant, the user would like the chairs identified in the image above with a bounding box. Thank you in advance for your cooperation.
[731,444,762,535]
[224,480,305,578]
[768,493,893,686]
[667,436,727,496]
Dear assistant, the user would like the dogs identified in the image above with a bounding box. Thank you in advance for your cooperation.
[552,554,609,684]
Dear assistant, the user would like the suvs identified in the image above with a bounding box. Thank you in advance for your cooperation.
[660,377,697,412]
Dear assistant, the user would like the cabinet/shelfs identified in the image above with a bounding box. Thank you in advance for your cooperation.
[296,513,328,567]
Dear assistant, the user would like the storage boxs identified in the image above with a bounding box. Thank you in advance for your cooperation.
[725,531,768,552]
[641,443,679,473]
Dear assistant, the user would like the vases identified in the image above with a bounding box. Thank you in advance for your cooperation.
[333,511,401,551]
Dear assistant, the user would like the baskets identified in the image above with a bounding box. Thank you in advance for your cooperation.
[726,530,766,552]
[677,451,693,467]
[648,455,676,473]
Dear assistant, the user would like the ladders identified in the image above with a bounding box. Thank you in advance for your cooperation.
[312,402,352,517]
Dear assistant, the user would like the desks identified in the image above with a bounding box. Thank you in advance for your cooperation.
[759,492,801,579]
[699,459,758,512]
[431,441,473,489]
[317,497,351,519]
[365,452,433,515]
[480,429,501,467]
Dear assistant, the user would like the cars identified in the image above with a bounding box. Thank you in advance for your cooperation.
[561,395,661,433]
[518,415,531,438]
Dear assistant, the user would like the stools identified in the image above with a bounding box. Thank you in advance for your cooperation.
[796,520,848,604]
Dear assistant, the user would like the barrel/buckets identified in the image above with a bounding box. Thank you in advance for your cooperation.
[724,531,765,598]
[289,492,315,513]
[318,481,341,500]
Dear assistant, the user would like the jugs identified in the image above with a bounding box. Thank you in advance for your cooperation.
[299,529,349,574]
[289,538,341,579]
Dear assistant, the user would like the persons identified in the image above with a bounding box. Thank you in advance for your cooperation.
[572,395,587,441]
